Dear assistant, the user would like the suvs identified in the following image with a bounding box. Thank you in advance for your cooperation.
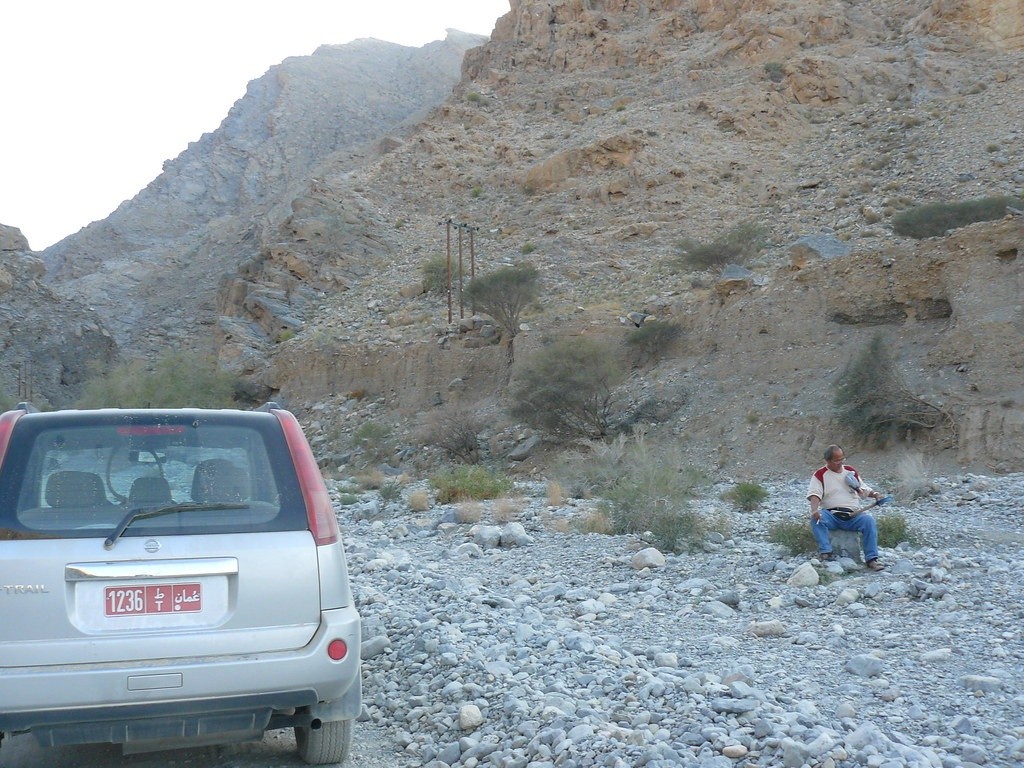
[0,402,362,765]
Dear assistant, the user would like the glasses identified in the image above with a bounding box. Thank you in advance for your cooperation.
[830,456,846,465]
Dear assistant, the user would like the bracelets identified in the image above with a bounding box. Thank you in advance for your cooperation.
[873,492,879,495]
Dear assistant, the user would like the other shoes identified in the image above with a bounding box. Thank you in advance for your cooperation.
[819,553,836,562]
[867,558,885,571]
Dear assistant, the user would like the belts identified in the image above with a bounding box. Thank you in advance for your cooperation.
[826,506,853,512]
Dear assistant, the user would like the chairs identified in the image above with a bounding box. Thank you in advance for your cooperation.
[234,468,251,502]
[80,472,115,506]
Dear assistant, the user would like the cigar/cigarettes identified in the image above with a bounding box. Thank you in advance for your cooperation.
[816,518,820,525]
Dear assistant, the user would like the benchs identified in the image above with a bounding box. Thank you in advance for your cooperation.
[17,457,280,529]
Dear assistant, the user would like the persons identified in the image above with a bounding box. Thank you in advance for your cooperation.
[806,444,888,571]
